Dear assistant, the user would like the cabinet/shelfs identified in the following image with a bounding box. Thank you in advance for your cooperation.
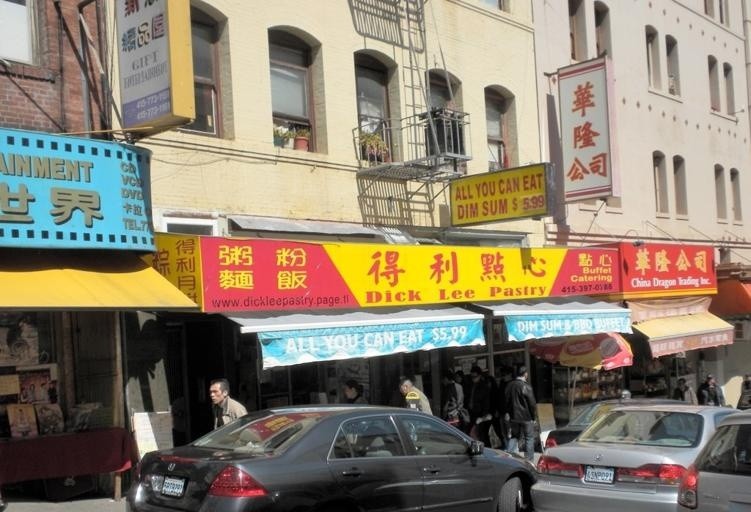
[551,362,624,418]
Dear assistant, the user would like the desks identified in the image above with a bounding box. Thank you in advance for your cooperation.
[0,427,133,501]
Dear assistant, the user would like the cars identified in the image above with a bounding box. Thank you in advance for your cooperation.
[126,403,539,510]
[532,404,751,511]
[544,398,697,451]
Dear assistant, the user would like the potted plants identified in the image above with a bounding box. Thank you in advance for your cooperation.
[358,129,387,159]
[295,128,312,150]
[274,128,296,149]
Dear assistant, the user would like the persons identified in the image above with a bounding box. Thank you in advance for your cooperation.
[19,379,57,403]
[697,373,726,407]
[208,377,248,431]
[672,377,698,405]
[441,362,537,463]
[343,380,369,405]
[398,378,433,416]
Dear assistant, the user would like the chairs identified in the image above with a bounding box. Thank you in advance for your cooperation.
[361,426,398,457]
[236,429,264,446]
